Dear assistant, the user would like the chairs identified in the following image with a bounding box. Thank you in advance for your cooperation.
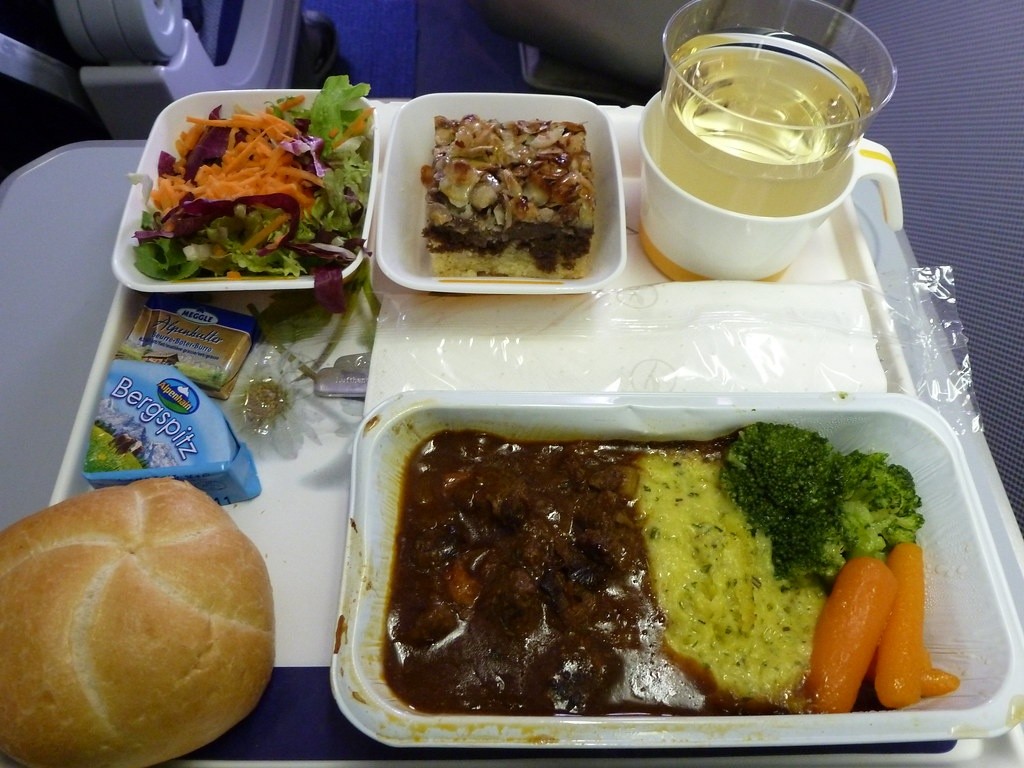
[0,0,338,138]
[471,1,859,104]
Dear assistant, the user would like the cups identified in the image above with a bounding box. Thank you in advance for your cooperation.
[655,0,898,218]
[638,91,903,288]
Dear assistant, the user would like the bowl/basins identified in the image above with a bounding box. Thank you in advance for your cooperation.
[110,89,377,292]
[370,92,629,295]
[332,390,1024,751]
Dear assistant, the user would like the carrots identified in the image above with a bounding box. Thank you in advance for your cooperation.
[802,544,961,713]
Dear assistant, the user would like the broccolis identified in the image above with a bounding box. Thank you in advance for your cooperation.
[717,420,927,586]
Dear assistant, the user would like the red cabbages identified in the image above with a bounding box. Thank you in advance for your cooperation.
[131,105,373,314]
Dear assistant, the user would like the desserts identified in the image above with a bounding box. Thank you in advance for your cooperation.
[418,113,596,280]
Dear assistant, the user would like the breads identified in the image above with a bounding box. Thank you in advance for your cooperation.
[0,476,276,768]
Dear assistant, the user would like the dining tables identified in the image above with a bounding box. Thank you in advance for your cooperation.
[0,138,1024,767]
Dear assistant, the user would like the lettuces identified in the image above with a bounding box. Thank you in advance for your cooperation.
[132,74,373,275]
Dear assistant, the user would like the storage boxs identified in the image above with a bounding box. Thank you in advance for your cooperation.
[118,299,261,400]
